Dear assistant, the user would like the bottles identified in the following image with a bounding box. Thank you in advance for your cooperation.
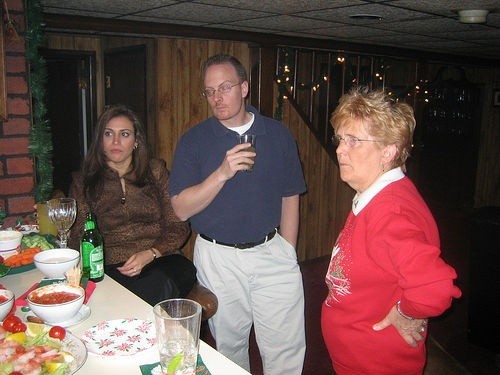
[80,212,104,282]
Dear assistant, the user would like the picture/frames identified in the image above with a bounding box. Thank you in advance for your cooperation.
[492,89,500,106]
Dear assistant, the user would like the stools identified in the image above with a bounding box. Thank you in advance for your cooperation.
[168,284,219,323]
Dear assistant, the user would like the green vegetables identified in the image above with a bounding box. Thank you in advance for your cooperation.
[22,235,55,251]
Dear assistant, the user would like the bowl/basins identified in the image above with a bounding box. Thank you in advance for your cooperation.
[33,248,80,279]
[0,230,22,252]
[0,289,15,323]
[27,283,85,324]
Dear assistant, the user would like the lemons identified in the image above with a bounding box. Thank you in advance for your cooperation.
[26,323,42,337]
[3,332,27,344]
[168,355,184,375]
[45,359,67,373]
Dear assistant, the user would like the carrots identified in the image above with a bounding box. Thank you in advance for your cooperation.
[3,247,42,267]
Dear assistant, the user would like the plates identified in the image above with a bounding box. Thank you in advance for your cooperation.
[0,321,88,375]
[43,305,91,329]
[81,318,158,355]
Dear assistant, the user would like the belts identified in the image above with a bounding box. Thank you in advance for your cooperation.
[199,230,276,249]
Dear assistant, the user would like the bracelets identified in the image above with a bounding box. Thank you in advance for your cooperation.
[150,247,156,261]
[397,300,412,320]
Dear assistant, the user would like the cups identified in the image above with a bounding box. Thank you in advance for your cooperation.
[235,135,257,172]
[152,299,203,375]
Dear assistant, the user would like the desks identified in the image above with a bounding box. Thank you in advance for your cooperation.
[0,231,252,375]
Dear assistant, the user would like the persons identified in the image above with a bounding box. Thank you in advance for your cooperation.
[169,54,306,375]
[321,85,462,375]
[65,105,196,312]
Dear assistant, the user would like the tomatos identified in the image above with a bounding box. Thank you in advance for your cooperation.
[2,316,27,333]
[48,326,66,340]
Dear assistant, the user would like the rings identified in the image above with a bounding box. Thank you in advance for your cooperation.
[420,326,425,334]
[134,267,136,272]
[422,319,427,325]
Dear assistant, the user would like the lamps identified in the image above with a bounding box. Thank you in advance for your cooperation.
[457,9,489,23]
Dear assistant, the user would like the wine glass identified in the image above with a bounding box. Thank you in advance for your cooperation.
[46,198,78,248]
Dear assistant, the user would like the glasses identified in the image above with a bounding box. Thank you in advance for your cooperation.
[330,136,376,147]
[202,83,241,97]
